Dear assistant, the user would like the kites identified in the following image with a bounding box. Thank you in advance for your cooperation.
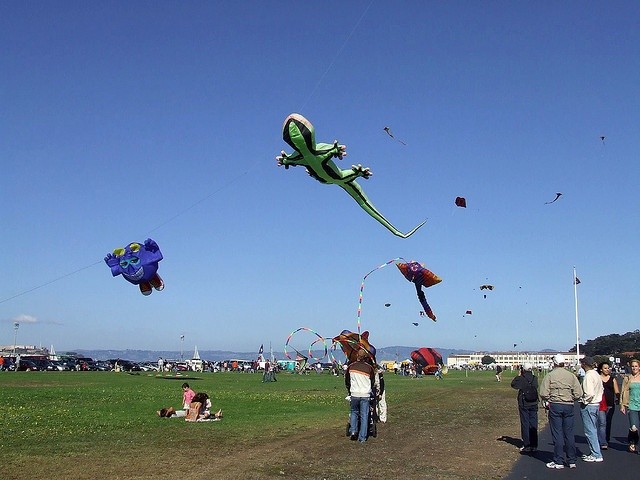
[455,195,467,208]
[547,192,563,206]
[356,256,445,345]
[283,327,376,372]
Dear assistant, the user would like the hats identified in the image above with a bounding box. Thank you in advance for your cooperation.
[553,354,566,365]
[579,357,596,368]
[522,362,532,371]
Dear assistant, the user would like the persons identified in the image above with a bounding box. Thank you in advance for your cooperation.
[538,354,582,470]
[595,362,619,446]
[510,361,539,452]
[344,348,375,444]
[435,365,445,382]
[618,358,640,453]
[261,359,278,383]
[161,406,203,419]
[571,354,605,463]
[496,365,504,382]
[180,382,193,410]
[190,392,223,419]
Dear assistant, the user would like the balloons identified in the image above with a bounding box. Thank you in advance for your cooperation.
[102,238,165,297]
[270,107,430,241]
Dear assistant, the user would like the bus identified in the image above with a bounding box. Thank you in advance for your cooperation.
[1,355,52,371]
[176,363,188,370]
[228,359,259,371]
[192,360,220,372]
[152,361,177,372]
[277,361,301,370]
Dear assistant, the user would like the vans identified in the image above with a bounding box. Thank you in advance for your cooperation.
[109,359,140,372]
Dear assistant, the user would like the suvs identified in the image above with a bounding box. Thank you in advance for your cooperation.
[76,358,96,370]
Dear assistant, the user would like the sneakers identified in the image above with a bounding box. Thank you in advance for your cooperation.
[582,453,604,462]
[519,446,532,455]
[545,460,564,470]
[530,446,538,454]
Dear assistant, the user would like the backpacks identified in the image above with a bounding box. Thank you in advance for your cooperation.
[523,374,540,402]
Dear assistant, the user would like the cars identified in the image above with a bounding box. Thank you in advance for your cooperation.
[96,364,108,370]
[47,360,71,371]
[139,363,157,371]
